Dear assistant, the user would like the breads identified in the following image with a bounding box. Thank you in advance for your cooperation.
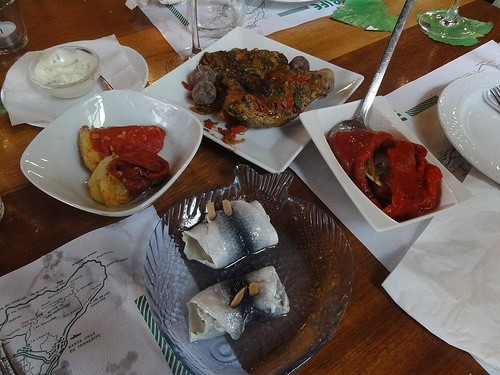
[77,124,105,173]
[88,154,133,207]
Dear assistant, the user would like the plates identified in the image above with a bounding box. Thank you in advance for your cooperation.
[20,90,203,216]
[1,40,149,128]
[298,95,475,233]
[144,163,355,375]
[437,71,500,184]
[143,26,365,175]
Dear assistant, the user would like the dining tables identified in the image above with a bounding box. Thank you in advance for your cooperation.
[0,1,500,375]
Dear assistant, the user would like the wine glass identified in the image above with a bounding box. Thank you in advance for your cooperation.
[419,0,476,40]
[166,0,208,72]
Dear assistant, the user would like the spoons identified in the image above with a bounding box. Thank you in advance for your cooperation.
[330,0,415,135]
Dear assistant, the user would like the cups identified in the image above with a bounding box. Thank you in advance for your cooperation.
[0,0,29,54]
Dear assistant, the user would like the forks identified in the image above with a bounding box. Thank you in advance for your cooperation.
[490,84,500,105]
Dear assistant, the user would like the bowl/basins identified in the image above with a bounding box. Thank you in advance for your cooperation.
[29,45,100,99]
